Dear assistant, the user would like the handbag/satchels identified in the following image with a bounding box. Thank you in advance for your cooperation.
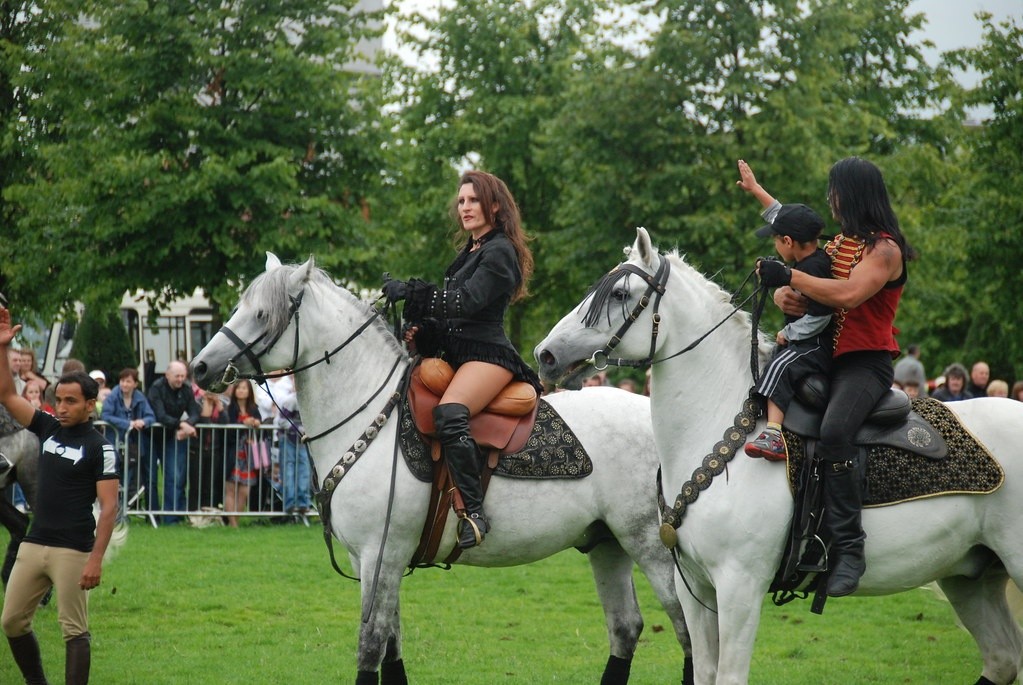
[251,418,269,469]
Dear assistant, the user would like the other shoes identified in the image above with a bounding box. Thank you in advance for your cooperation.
[40,586,53,605]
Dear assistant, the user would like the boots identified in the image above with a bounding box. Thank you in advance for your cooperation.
[814,456,867,597]
[8,631,47,685]
[432,403,491,546]
[65,631,91,685]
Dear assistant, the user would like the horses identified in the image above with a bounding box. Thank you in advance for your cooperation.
[192,250,694,685]
[532,227,1022,685]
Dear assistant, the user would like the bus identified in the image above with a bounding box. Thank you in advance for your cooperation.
[13,282,228,385]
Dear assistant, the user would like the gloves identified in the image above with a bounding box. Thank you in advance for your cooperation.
[382,279,405,304]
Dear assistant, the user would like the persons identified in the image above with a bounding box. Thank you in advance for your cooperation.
[583,371,608,387]
[891,344,975,402]
[968,361,990,399]
[645,368,650,396]
[0,349,112,528]
[190,369,318,527]
[986,379,1009,398]
[618,376,640,392]
[540,379,566,396]
[0,303,119,685]
[1012,381,1023,402]
[753,157,920,598]
[736,159,832,461]
[382,172,544,550]
[146,360,198,525]
[101,368,156,523]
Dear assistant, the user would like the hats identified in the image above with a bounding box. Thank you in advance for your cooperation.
[89,370,105,381]
[754,203,826,239]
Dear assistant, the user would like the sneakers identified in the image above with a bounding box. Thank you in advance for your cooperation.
[745,431,786,461]
